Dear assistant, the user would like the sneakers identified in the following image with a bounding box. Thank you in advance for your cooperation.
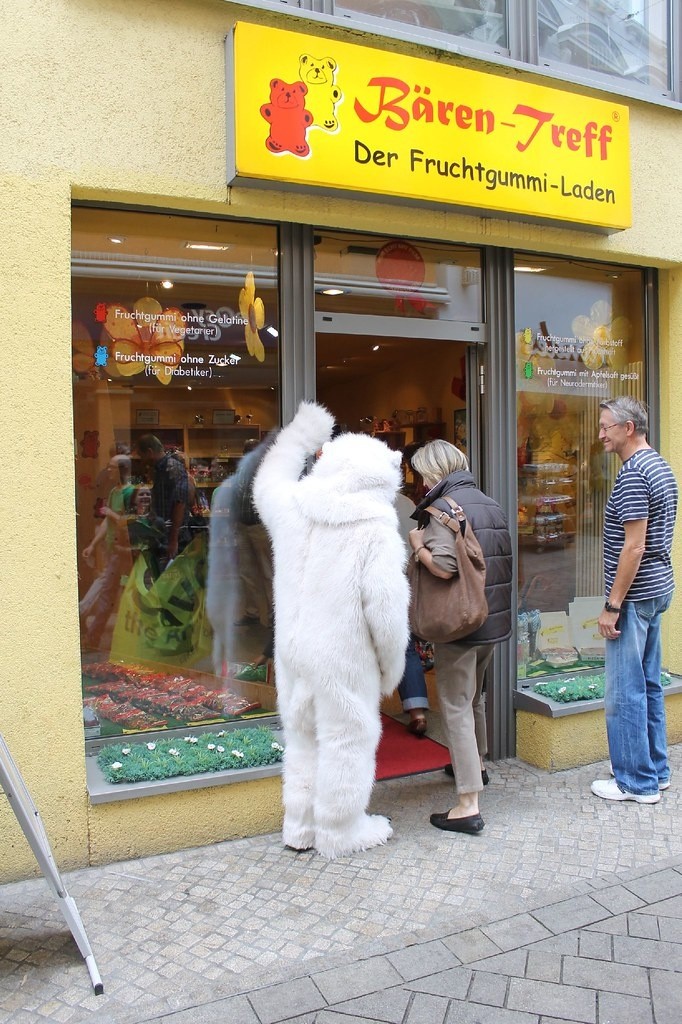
[591,778,660,803]
[610,764,670,790]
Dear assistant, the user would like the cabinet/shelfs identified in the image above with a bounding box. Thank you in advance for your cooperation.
[400,421,447,489]
[375,431,408,487]
[518,469,575,543]
[112,423,262,557]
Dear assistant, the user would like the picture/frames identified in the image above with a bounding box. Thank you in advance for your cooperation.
[453,409,466,456]
[211,409,235,425]
[136,408,159,425]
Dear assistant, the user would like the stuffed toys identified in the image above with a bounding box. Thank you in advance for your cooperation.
[253,400,413,861]
[205,427,281,689]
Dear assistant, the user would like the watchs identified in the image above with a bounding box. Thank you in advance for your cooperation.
[415,546,426,562]
[604,602,621,613]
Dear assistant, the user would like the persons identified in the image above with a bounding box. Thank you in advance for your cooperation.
[409,441,513,832]
[79,434,275,671]
[391,493,431,734]
[591,397,679,804]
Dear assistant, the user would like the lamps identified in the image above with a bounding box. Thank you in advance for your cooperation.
[368,343,379,351]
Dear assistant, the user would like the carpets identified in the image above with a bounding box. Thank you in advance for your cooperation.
[378,711,453,781]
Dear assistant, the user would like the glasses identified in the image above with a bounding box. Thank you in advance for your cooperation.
[108,465,124,470]
[600,423,619,433]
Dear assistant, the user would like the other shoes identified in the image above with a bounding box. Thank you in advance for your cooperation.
[430,808,485,832]
[406,719,427,733]
[81,639,99,650]
[445,764,489,785]
[234,614,261,626]
[156,636,193,655]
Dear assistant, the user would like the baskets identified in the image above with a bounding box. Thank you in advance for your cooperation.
[518,609,540,632]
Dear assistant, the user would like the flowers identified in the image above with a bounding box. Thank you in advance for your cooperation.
[96,727,286,784]
[533,668,673,704]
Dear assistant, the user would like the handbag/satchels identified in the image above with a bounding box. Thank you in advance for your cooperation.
[406,497,489,643]
